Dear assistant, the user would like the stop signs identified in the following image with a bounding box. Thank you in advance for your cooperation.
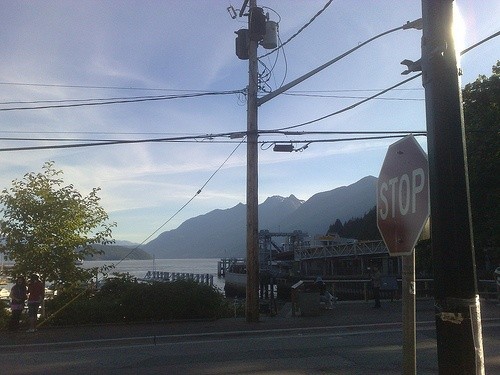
[374,134,432,258]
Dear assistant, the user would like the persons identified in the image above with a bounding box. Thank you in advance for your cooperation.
[370,265,382,307]
[10,276,32,331]
[26,274,45,332]
